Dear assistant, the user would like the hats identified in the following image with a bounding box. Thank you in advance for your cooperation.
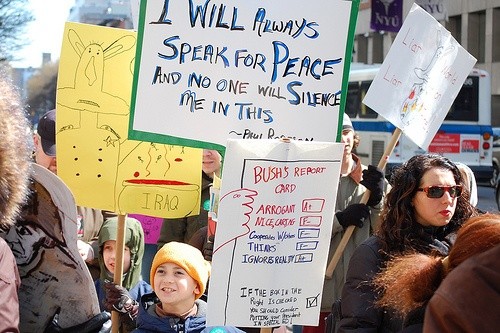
[150,241,211,300]
[342,113,356,133]
[37,109,56,156]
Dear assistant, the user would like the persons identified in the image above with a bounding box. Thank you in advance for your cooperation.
[338,153,479,333]
[30,109,118,283]
[369,178,500,333]
[94,216,153,333]
[302,113,392,333]
[132,241,246,333]
[156,148,223,262]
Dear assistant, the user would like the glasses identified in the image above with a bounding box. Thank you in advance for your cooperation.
[417,185,463,199]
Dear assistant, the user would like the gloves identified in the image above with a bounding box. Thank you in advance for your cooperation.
[103,281,139,323]
[360,164,384,206]
[336,203,372,228]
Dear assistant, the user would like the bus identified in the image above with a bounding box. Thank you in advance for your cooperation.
[342,66,493,177]
